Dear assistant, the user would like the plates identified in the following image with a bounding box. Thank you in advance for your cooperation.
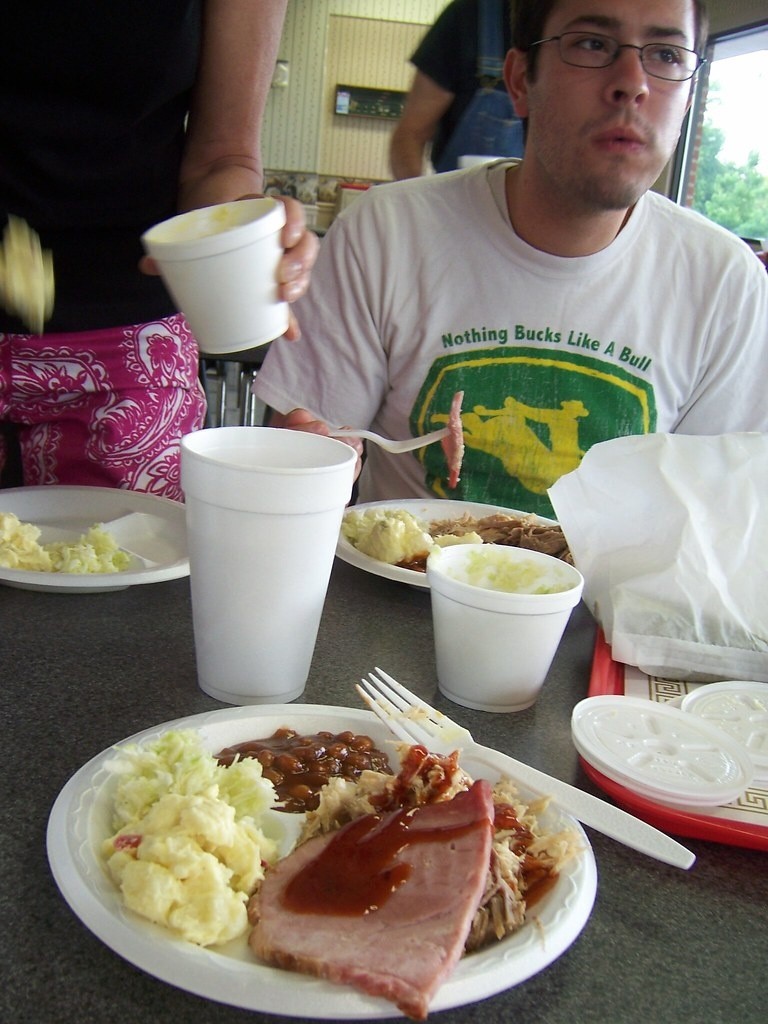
[335,499,560,590]
[45,705,599,1018]
[0,484,190,592]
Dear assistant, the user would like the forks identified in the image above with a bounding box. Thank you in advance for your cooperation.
[325,424,453,454]
[355,666,696,871]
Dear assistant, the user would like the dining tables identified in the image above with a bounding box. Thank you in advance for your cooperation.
[0,556,768,1024]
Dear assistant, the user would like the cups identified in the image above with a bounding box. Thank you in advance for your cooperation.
[180,426,358,707]
[140,198,289,354]
[427,543,585,713]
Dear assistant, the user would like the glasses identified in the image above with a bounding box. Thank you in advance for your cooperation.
[526,31,709,82]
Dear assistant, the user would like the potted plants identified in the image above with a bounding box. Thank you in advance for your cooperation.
[295,179,318,227]
[317,184,336,228]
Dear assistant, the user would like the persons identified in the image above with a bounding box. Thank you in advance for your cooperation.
[250,0,768,598]
[0,0,319,504]
